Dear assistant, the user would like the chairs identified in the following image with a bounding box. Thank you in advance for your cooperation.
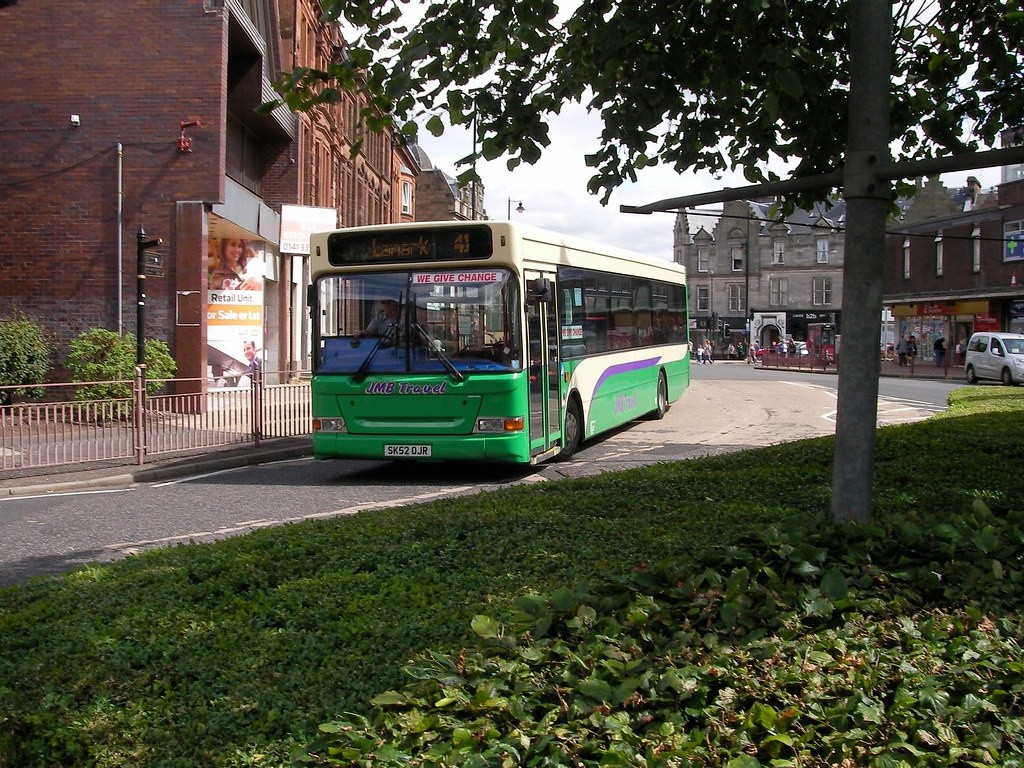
[585,309,670,350]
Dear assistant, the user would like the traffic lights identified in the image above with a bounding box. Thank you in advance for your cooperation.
[724,324,730,339]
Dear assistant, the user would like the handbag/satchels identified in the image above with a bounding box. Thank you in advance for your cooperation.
[747,355,752,362]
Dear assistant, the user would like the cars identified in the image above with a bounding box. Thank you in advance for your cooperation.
[964,332,1024,387]
[756,341,808,360]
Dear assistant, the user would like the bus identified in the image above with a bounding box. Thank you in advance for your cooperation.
[305,219,690,466]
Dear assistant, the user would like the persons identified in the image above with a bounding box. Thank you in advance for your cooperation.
[353,292,412,345]
[697,339,715,364]
[211,237,263,290]
[244,340,262,377]
[769,341,777,355]
[689,338,693,354]
[933,337,945,367]
[779,339,789,354]
[896,336,918,366]
[788,338,796,353]
[953,333,967,366]
[728,340,760,364]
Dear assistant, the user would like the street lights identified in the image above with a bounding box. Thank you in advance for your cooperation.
[508,197,526,220]
